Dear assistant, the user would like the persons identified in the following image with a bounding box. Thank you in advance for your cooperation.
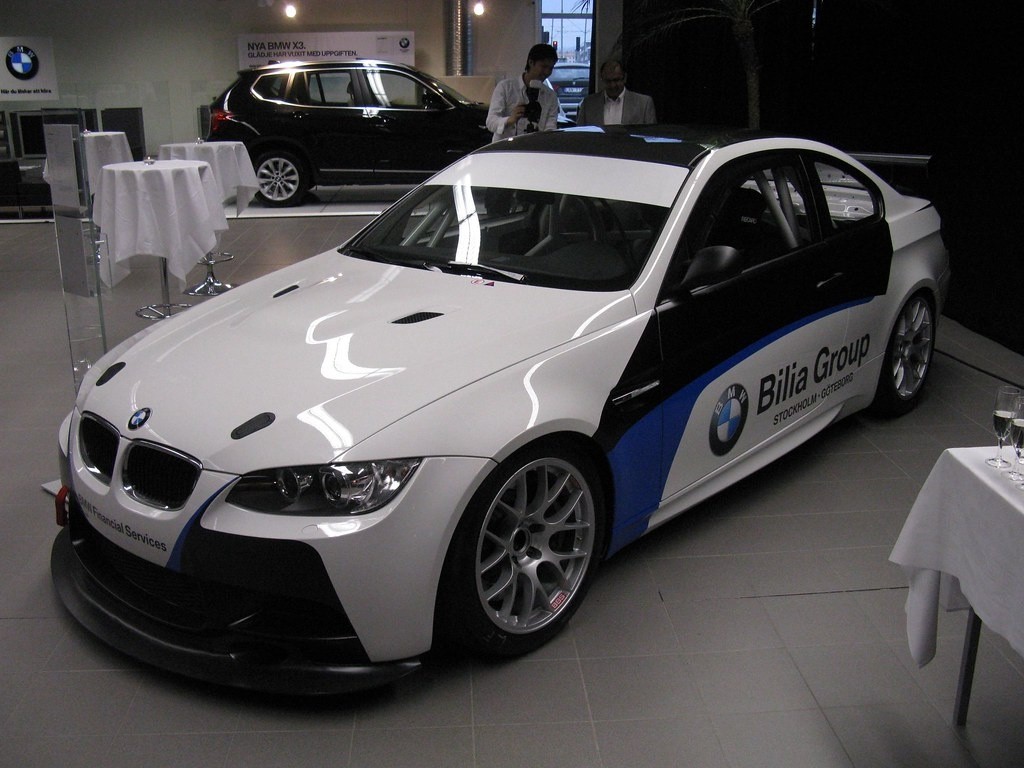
[577,60,657,127]
[484,44,559,215]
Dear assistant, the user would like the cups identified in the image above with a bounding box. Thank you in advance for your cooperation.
[194,136,204,145]
[143,153,155,166]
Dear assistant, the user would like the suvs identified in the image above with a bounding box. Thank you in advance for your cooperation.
[197,60,495,208]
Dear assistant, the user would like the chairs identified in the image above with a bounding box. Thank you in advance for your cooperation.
[524,197,605,260]
[702,190,769,258]
[0,158,50,224]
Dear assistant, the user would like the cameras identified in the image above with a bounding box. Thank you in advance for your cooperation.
[519,80,543,123]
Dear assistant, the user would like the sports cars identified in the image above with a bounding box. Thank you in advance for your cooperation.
[38,122,954,693]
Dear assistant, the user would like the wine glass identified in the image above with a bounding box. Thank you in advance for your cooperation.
[1000,397,1024,481]
[985,386,1021,468]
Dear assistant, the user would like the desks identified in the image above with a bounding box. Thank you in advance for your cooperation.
[43,131,134,197]
[890,445,1024,726]
[91,159,231,320]
[158,141,262,297]
